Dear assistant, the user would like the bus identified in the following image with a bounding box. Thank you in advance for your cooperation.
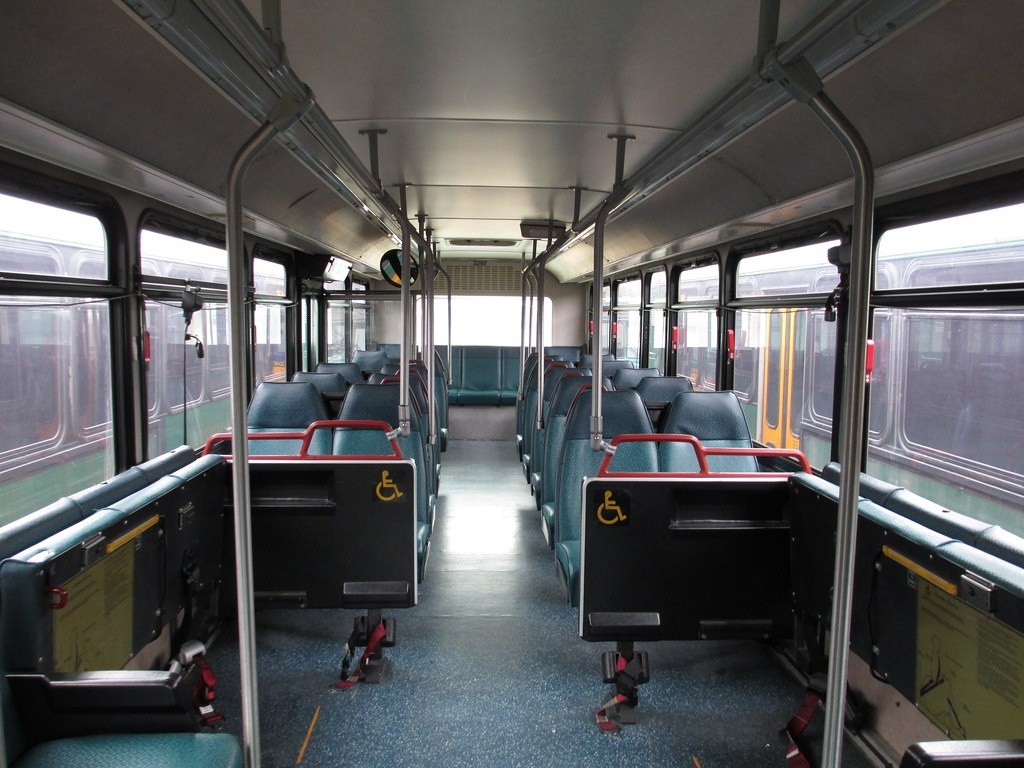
[0,231,319,534]
[634,237,1024,545]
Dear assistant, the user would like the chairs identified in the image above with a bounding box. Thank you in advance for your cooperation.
[0,662,245,767]
[245,340,761,610]
